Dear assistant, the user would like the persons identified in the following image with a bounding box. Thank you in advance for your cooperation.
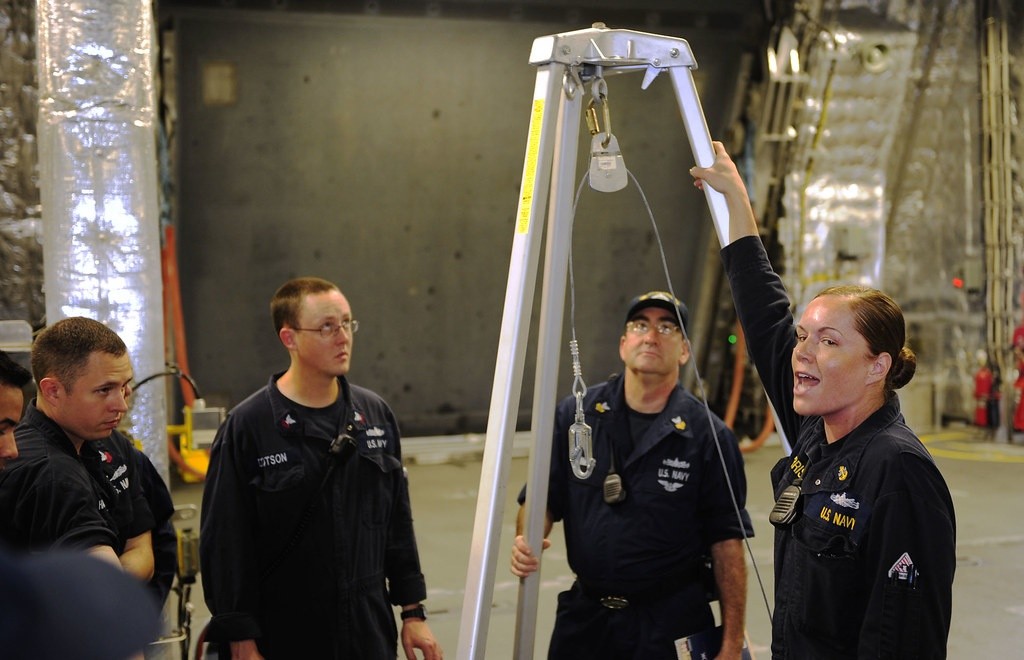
[690,142,956,660]
[200,277,445,660]
[509,292,755,660]
[0,317,178,660]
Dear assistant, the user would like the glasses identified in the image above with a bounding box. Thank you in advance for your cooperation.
[294,319,360,338]
[625,319,683,337]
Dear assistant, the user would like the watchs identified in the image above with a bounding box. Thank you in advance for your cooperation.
[401,603,428,622]
